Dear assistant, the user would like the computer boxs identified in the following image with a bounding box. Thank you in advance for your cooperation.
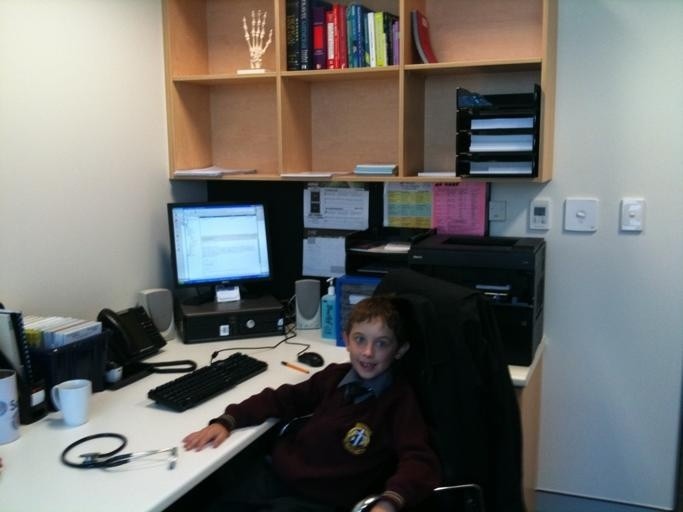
[173,291,287,344]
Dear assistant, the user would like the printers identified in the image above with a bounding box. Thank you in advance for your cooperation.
[410,233,546,367]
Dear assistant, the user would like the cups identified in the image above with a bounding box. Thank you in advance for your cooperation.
[51,379,92,426]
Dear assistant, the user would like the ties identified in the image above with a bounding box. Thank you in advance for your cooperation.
[344,383,367,408]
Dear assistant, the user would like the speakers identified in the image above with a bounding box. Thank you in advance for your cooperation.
[295,278,321,329]
[139,288,176,341]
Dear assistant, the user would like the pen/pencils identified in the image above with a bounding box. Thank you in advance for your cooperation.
[282,361,310,374]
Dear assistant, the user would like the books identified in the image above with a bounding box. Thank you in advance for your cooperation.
[173,165,258,177]
[0,308,102,390]
[353,164,399,175]
[410,9,438,64]
[286,0,400,71]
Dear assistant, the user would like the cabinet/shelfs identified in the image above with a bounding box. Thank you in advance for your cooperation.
[161,0,560,183]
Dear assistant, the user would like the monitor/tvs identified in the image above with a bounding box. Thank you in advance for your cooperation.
[166,200,274,306]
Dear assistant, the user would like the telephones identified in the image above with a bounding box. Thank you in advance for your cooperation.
[97,306,167,362]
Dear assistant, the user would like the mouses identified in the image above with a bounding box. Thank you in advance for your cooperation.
[298,351,323,366]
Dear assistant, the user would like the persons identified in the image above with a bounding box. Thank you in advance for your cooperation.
[183,298,440,512]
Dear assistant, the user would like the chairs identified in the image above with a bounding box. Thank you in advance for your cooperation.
[352,272,525,510]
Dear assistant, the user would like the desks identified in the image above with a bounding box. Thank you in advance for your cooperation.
[0,335,547,512]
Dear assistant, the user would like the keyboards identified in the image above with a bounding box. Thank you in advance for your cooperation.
[148,352,269,412]
